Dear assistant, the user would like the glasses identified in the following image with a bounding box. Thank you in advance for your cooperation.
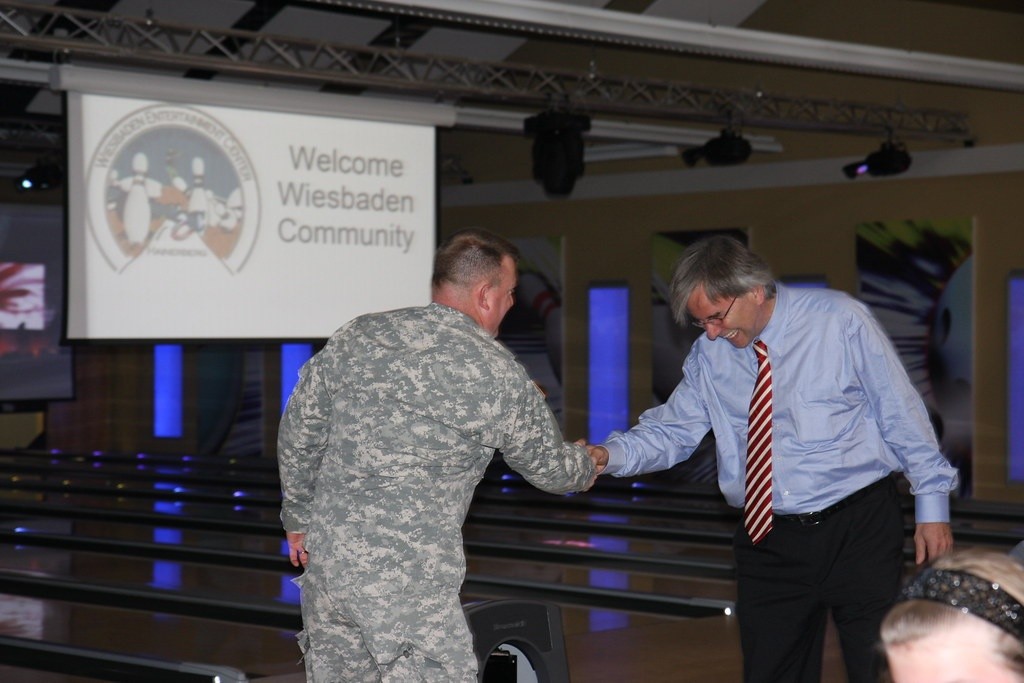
[690,293,739,329]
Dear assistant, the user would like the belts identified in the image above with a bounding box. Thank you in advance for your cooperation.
[773,473,894,529]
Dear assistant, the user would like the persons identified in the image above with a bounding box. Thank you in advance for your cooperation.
[881,551,1023,683]
[585,236,960,683]
[277,230,599,683]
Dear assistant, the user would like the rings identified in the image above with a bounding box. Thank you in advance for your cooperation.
[299,551,308,555]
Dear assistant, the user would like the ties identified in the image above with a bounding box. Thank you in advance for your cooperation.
[744,340,772,545]
